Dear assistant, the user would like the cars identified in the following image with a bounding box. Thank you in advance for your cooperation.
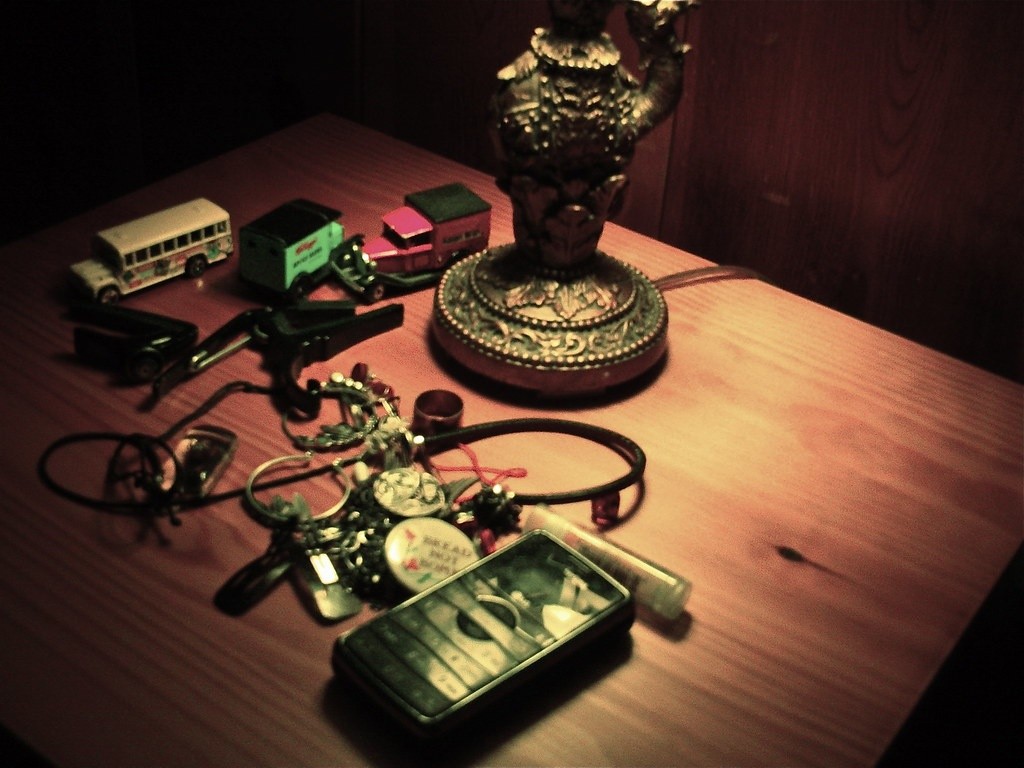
[334,181,492,304]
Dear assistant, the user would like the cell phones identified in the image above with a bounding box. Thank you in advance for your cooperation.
[330,528,634,746]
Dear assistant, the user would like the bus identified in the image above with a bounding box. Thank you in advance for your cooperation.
[71,198,235,304]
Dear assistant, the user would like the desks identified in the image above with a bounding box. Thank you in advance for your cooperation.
[0,113,1024,768]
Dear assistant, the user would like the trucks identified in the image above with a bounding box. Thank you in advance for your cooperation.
[240,199,344,300]
